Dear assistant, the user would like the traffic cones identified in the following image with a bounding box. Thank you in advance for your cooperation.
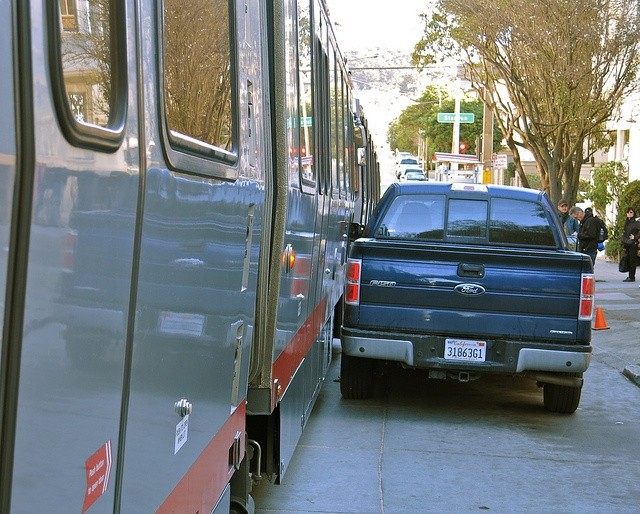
[592,307,610,330]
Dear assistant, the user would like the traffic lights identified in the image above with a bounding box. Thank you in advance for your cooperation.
[460,143,465,151]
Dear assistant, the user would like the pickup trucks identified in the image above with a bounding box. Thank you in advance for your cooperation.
[396,158,422,179]
[339,180,596,414]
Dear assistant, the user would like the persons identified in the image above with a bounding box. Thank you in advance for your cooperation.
[621,205,640,282]
[585,207,592,213]
[556,199,581,251]
[569,206,598,266]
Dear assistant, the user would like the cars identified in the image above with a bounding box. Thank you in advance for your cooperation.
[401,171,427,180]
[395,151,412,164]
[400,168,424,180]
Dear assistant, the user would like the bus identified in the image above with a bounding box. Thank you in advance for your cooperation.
[0,0,370,513]
[349,96,381,242]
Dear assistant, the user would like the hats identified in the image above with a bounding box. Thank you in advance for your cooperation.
[558,199,568,206]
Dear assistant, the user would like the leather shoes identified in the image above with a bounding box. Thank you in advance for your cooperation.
[623,277,635,281]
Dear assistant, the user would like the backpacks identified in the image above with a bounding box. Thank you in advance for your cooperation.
[584,216,608,242]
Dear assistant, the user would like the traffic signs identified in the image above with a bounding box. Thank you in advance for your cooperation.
[436,112,474,122]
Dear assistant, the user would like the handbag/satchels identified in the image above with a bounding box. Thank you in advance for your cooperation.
[619,251,629,272]
[621,236,634,245]
